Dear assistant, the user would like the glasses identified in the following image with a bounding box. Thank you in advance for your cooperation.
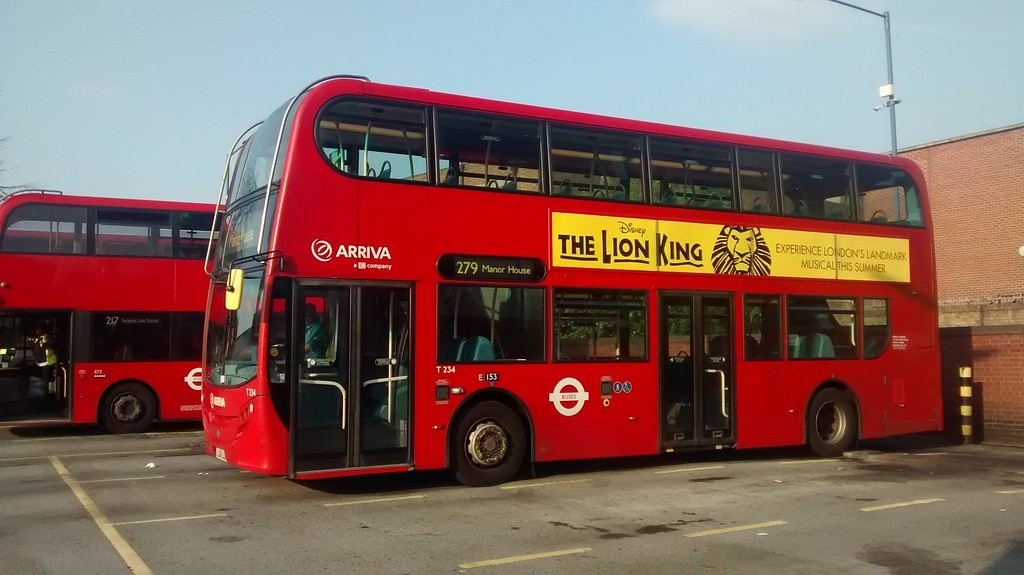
[304,311,313,316]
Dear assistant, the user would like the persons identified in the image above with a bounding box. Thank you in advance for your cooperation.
[304,303,327,359]
[27,325,59,398]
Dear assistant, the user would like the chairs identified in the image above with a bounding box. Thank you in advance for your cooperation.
[444,336,497,363]
[560,338,590,361]
[709,333,852,362]
[667,357,689,383]
[365,155,892,227]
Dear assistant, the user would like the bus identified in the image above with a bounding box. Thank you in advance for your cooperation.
[199,73,942,489]
[0,187,328,436]
